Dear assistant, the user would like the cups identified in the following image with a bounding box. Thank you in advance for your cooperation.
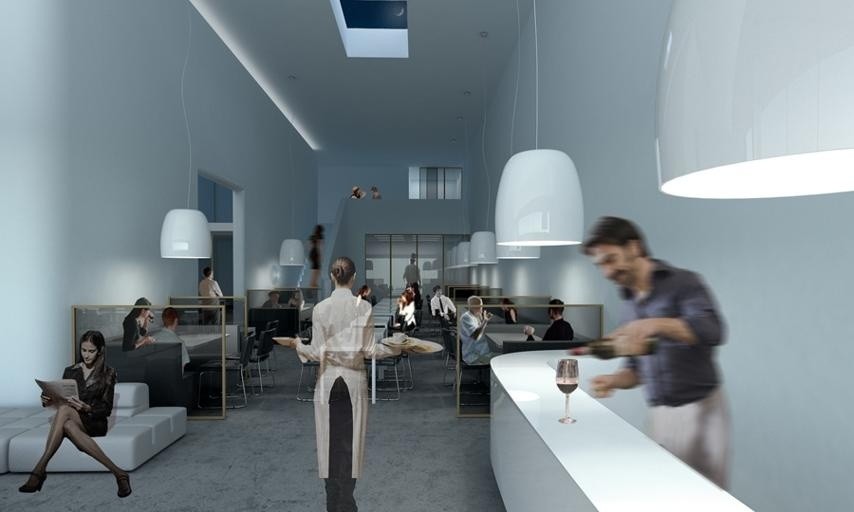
[392,332,408,343]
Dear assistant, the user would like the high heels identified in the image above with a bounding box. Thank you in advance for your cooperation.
[19,470,47,493]
[116,472,131,497]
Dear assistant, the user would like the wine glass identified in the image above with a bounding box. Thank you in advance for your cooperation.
[553,359,578,425]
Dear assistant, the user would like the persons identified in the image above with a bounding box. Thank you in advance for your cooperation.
[567,215,731,489]
[19,331,131,498]
[289,258,403,512]
[370,186,383,199]
[122,225,573,372]
[350,186,367,199]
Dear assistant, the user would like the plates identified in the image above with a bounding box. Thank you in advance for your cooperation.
[272,336,309,348]
[381,337,421,349]
[411,341,444,354]
[387,336,409,345]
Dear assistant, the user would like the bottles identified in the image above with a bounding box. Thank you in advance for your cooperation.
[568,333,658,360]
